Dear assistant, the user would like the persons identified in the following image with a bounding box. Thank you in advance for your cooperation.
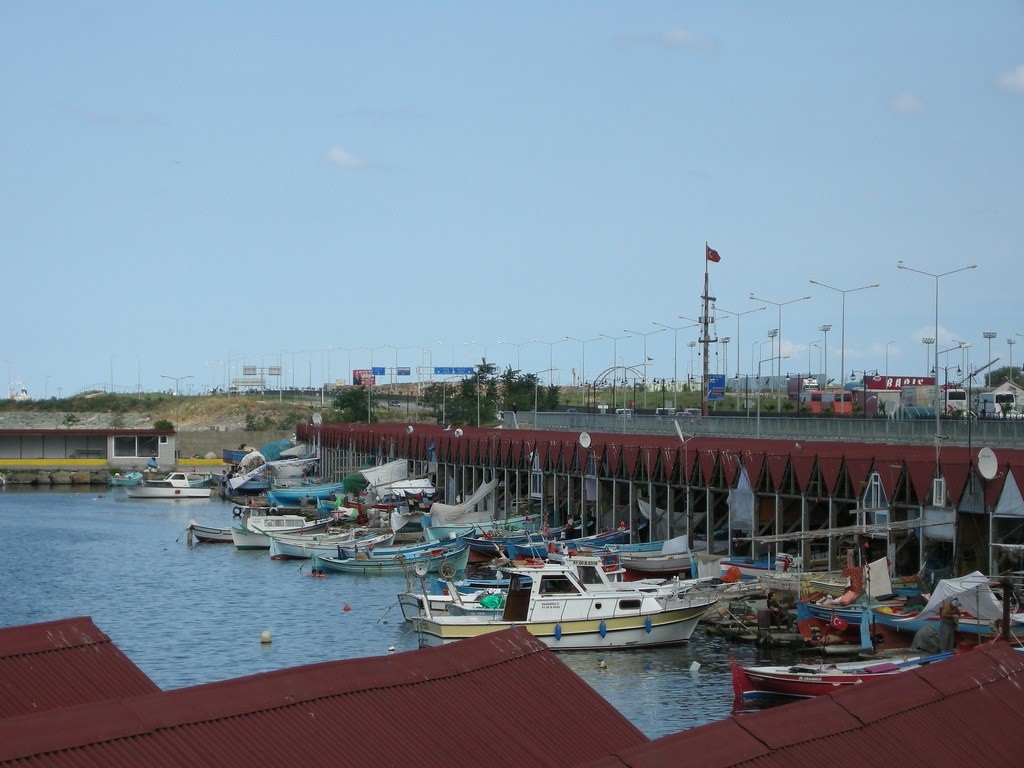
[766,592,794,625]
[939,600,960,654]
[353,371,362,384]
[566,515,574,539]
[299,495,311,506]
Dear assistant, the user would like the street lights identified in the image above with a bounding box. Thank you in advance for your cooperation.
[622,363,653,434]
[849,368,881,434]
[534,367,559,430]
[756,354,791,438]
[651,321,701,410]
[653,376,676,415]
[720,336,731,378]
[768,328,779,399]
[819,324,833,391]
[814,344,823,391]
[982,331,998,392]
[530,338,569,387]
[710,305,767,411]
[809,279,881,416]
[598,333,634,409]
[784,372,813,416]
[734,372,760,418]
[897,264,979,441]
[623,328,667,410]
[749,295,811,413]
[226,339,522,428]
[686,341,698,379]
[565,335,603,406]
[930,363,963,419]
[501,339,536,377]
[962,343,974,392]
[922,337,935,377]
[159,374,195,466]
[1007,339,1017,381]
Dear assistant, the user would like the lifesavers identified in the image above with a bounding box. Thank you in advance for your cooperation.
[232,507,241,516]
[269,507,279,516]
[524,563,545,568]
[601,552,619,572]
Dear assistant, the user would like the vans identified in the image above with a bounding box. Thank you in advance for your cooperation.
[974,391,1016,416]
[938,388,968,412]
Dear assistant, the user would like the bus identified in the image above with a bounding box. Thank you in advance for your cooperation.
[799,388,854,416]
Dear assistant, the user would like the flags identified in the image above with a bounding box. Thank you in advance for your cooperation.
[708,248,721,262]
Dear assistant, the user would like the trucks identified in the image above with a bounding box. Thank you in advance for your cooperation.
[786,373,820,401]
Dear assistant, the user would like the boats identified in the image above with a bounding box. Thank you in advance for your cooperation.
[107,436,1024,714]
